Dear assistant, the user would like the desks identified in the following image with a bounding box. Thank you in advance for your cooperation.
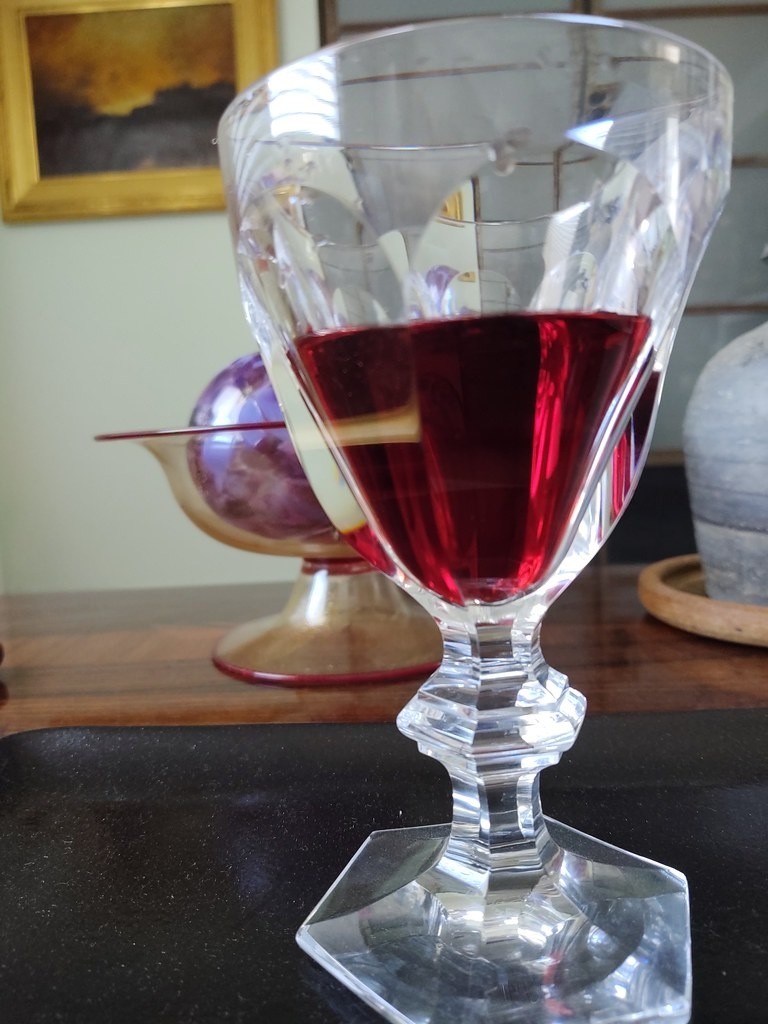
[0,569,768,1024]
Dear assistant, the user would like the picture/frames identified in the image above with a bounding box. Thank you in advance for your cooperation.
[0,0,281,226]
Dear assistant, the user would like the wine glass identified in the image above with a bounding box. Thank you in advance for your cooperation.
[218,11,736,1024]
